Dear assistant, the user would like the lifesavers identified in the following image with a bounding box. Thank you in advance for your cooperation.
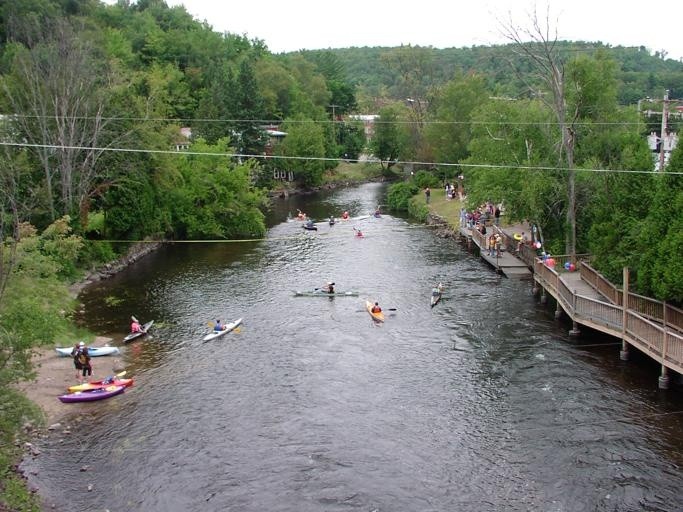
[514,233,522,240]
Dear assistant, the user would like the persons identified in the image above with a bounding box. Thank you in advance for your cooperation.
[70,344,82,378]
[544,253,555,270]
[371,302,381,314]
[423,187,431,207]
[432,286,439,295]
[515,223,540,253]
[327,282,335,294]
[81,347,92,379]
[457,199,504,258]
[213,319,222,331]
[441,180,457,201]
[536,251,549,265]
[130,319,143,333]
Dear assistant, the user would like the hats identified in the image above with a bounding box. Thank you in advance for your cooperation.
[493,234,499,237]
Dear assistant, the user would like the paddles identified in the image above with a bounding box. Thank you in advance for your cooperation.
[315,283,336,290]
[382,308,397,312]
[208,321,241,334]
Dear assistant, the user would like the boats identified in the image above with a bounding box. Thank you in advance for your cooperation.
[55,384,127,403]
[365,300,385,323]
[66,378,133,394]
[285,209,383,239]
[123,319,155,342]
[428,280,444,307]
[291,284,357,300]
[201,317,243,342]
[55,346,118,358]
